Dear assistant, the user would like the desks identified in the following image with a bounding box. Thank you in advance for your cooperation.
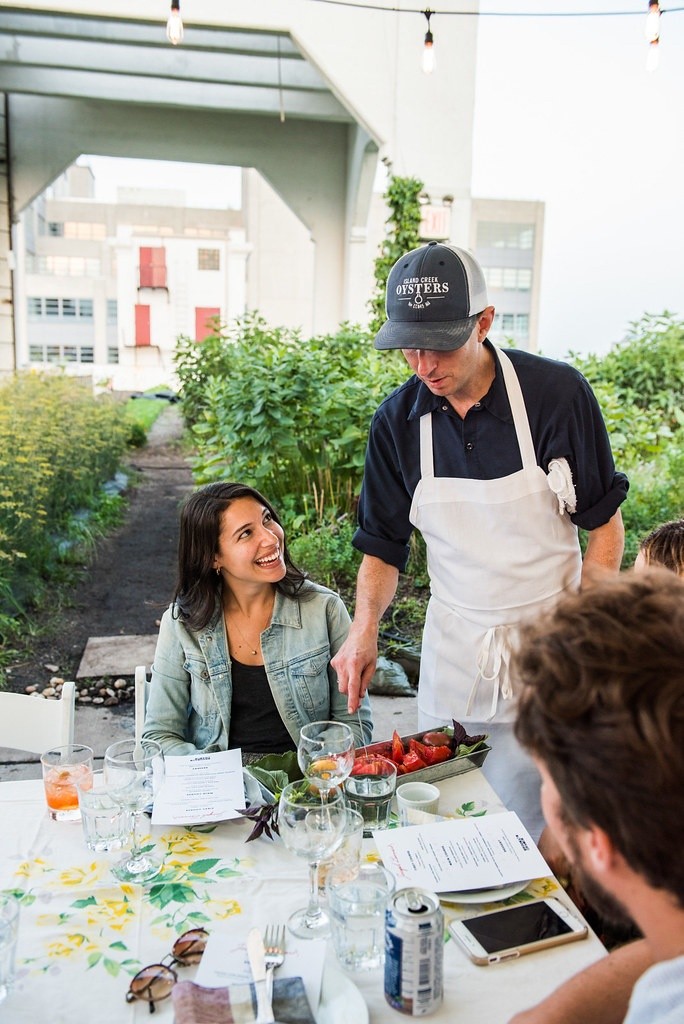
[0,769,608,1024]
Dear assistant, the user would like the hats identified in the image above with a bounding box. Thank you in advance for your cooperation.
[373,242,489,350]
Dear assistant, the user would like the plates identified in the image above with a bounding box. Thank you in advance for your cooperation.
[197,960,370,1024]
[438,879,533,905]
[331,725,493,783]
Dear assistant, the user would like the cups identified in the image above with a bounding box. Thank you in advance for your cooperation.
[75,767,137,853]
[324,859,396,972]
[396,782,441,827]
[340,758,397,839]
[304,806,365,900]
[39,743,94,823]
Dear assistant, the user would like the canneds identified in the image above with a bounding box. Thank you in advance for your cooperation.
[384,887,444,1016]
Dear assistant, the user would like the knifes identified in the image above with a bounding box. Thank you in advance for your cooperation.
[246,927,275,1024]
[356,697,367,758]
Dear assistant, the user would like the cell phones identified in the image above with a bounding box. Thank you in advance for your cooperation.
[449,896,591,967]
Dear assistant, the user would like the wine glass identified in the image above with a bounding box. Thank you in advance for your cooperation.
[296,721,356,832]
[103,739,166,884]
[277,777,348,941]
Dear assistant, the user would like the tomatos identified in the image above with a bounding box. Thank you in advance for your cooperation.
[339,730,452,778]
[306,759,344,797]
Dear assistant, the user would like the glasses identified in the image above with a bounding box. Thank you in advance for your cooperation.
[126,928,209,1012]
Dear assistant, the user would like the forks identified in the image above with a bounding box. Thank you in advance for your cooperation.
[260,924,286,1006]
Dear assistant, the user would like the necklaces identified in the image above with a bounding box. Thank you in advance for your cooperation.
[225,602,272,656]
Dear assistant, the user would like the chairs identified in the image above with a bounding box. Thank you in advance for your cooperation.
[0,666,150,758]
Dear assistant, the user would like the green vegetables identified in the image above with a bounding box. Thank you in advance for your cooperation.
[442,718,487,759]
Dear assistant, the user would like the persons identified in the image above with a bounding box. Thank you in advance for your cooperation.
[503,566,683,1024]
[534,519,684,958]
[329,241,629,851]
[139,481,374,770]
